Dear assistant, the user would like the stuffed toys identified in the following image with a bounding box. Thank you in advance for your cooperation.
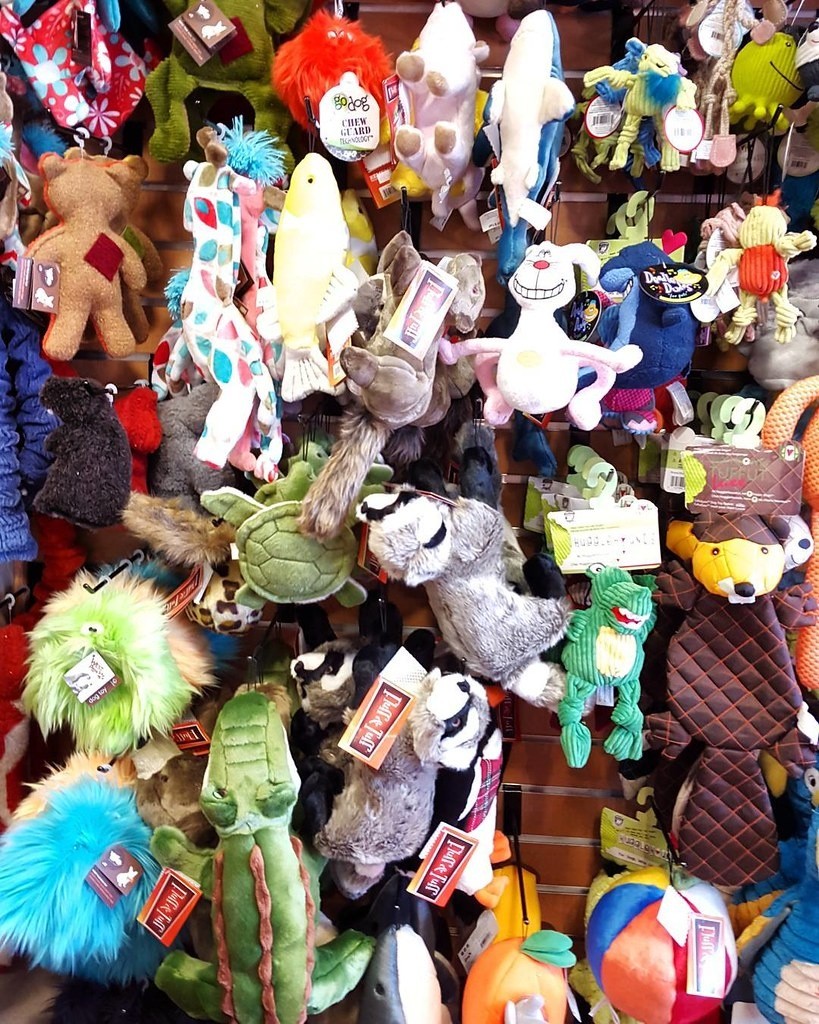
[24,150,149,361]
[0,1,819,1024]
